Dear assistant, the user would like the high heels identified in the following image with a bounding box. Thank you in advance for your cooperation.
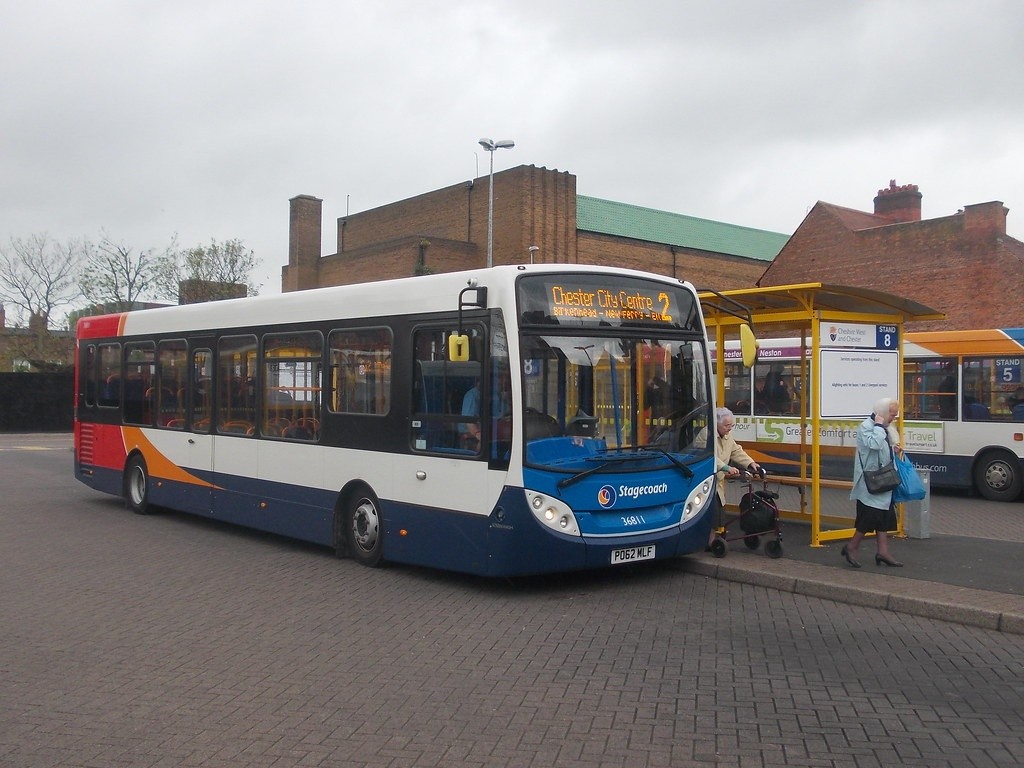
[841,544,861,567]
[875,553,904,567]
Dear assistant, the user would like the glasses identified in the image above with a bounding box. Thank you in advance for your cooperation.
[724,424,735,428]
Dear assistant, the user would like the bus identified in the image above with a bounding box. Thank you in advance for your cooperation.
[74,262,761,574]
[642,326,1024,501]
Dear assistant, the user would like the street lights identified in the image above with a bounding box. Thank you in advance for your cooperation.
[529,246,540,264]
[478,137,515,268]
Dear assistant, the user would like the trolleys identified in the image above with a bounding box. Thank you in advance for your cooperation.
[711,467,785,560]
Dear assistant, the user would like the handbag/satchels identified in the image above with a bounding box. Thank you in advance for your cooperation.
[893,450,927,503]
[740,491,779,534]
[864,461,901,494]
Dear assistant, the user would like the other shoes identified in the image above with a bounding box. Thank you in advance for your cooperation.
[705,545,712,551]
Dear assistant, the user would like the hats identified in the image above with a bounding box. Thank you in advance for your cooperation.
[494,360,510,375]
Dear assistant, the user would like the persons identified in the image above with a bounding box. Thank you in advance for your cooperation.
[457,377,508,452]
[841,398,905,568]
[695,407,761,551]
